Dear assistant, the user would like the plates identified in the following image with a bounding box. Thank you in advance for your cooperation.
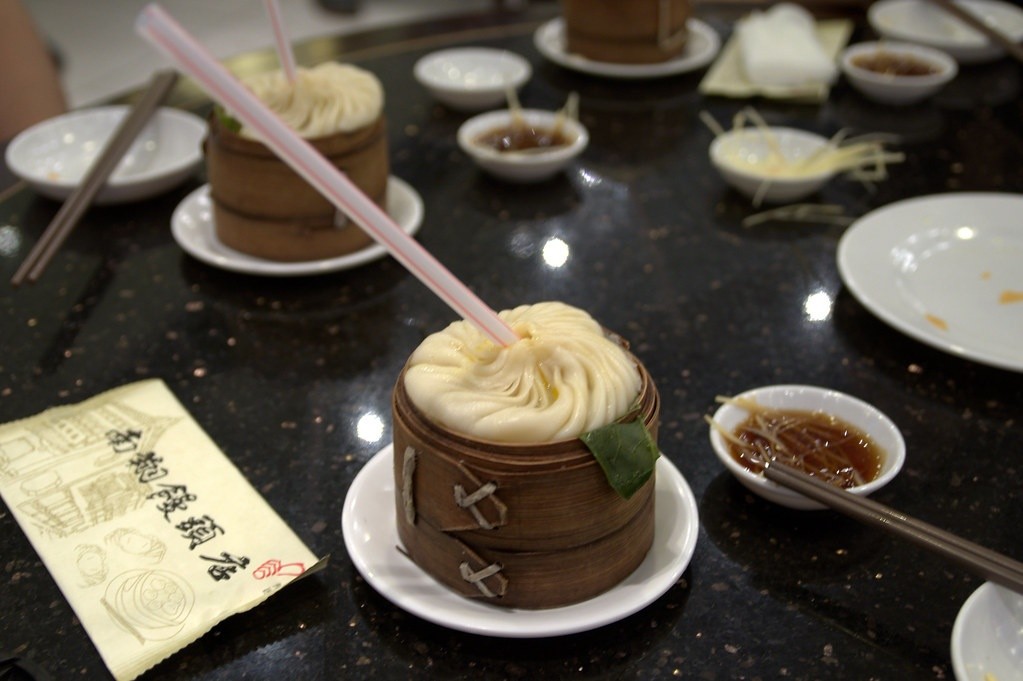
[171,178,427,275]
[341,442,700,639]
[709,384,906,510]
[708,127,843,203]
[455,109,589,183]
[836,41,959,106]
[950,581,1023,681]
[533,17,720,77]
[411,47,532,111]
[5,106,210,203]
[834,191,1023,372]
[697,18,854,104]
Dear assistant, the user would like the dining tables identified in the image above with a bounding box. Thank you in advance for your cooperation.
[0,0,1023,681]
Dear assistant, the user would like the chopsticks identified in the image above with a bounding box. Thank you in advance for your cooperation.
[931,0,1023,60]
[762,461,1023,594]
[9,70,178,287]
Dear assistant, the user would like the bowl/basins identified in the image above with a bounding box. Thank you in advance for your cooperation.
[867,0,1023,65]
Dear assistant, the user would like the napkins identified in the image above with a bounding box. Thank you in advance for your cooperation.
[732,3,837,89]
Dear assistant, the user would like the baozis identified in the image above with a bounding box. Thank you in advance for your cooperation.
[223,61,382,141]
[403,301,641,442]
